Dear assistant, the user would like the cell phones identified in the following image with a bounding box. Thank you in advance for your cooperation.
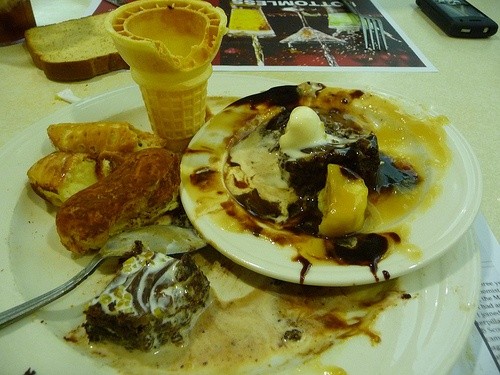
[416,0,498,38]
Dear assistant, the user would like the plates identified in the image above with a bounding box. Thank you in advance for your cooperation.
[179,83,482,287]
[1,75,481,374]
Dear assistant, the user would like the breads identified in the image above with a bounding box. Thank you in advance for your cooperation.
[24,12,130,83]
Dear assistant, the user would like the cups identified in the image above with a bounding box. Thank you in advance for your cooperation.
[227,3,275,37]
[326,6,363,28]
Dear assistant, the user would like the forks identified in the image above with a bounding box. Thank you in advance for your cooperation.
[344,0,388,50]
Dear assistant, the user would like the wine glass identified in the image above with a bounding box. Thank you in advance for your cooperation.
[279,7,348,43]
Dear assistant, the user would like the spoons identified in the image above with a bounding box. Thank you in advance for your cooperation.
[0,224,207,331]
